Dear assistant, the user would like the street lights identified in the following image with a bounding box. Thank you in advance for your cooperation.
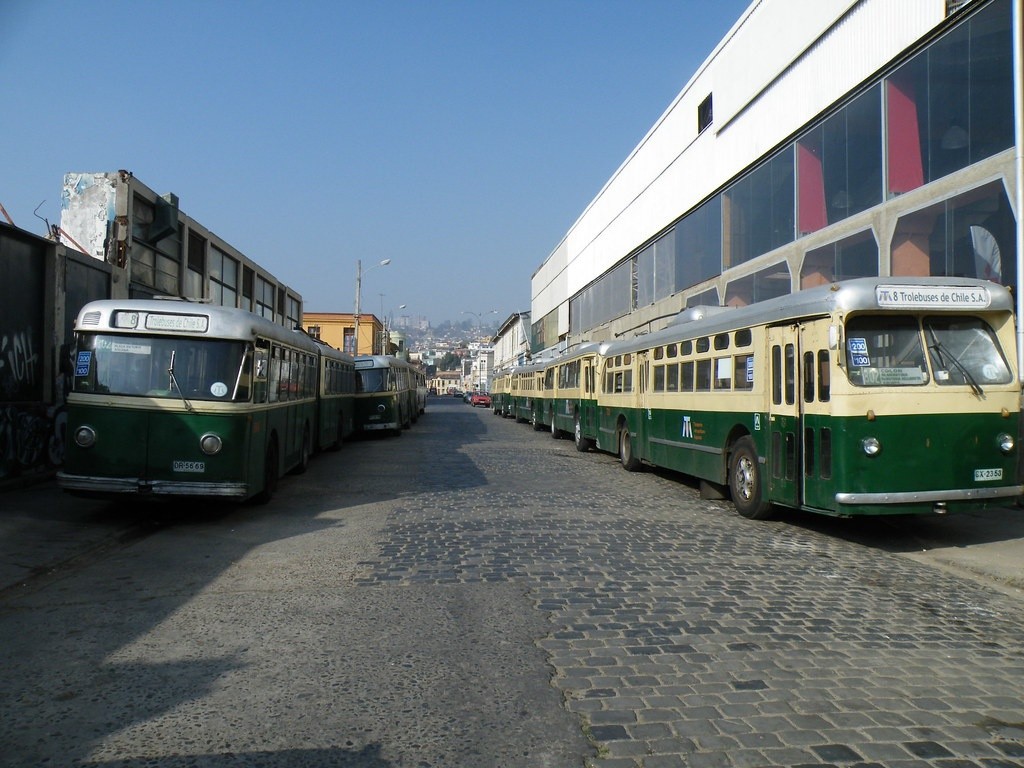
[381,305,407,356]
[353,258,390,359]
[461,310,499,395]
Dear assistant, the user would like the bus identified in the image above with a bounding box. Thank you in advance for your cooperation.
[352,354,429,436]
[541,342,622,453]
[52,297,318,504]
[310,336,359,452]
[593,276,1023,521]
[490,365,518,419]
[510,364,545,431]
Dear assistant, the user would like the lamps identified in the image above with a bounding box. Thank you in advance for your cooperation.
[941,121,969,148]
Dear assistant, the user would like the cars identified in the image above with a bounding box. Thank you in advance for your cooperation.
[470,391,492,407]
[428,387,438,396]
[447,386,473,404]
